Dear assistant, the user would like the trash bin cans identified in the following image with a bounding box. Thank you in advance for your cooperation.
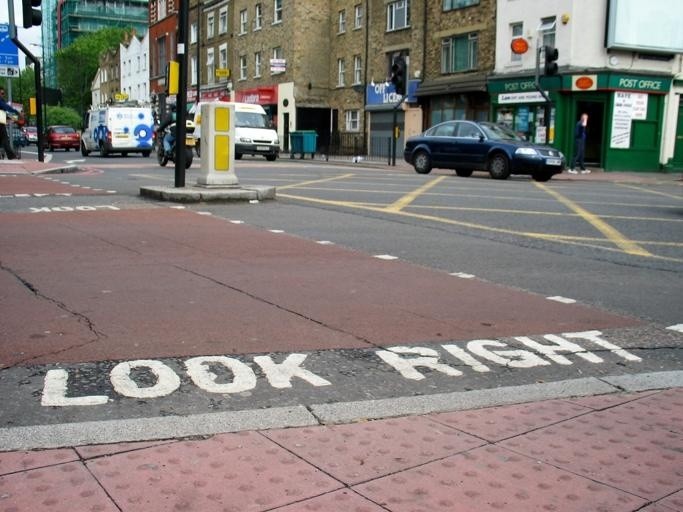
[289,130,316,159]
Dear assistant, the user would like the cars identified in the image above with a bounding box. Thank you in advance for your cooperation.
[403,120,565,182]
[43,126,80,152]
[25,127,38,144]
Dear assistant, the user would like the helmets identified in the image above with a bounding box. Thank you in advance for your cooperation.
[170,102,177,111]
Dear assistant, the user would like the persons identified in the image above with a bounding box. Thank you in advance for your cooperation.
[0,85,24,159]
[568,112,591,174]
[153,101,176,158]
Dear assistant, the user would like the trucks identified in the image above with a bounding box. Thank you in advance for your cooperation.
[81,102,156,157]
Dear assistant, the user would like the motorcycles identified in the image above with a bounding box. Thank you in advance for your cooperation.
[157,120,194,169]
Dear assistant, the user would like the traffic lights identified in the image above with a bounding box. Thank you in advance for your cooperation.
[392,56,406,95]
[544,45,558,77]
[23,0,43,29]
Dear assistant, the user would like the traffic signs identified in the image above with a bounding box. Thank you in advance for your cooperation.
[0,23,18,79]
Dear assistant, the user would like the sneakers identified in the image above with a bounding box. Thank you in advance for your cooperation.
[581,169,591,174]
[568,168,578,174]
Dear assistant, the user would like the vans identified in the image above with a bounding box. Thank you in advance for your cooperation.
[193,100,280,162]
